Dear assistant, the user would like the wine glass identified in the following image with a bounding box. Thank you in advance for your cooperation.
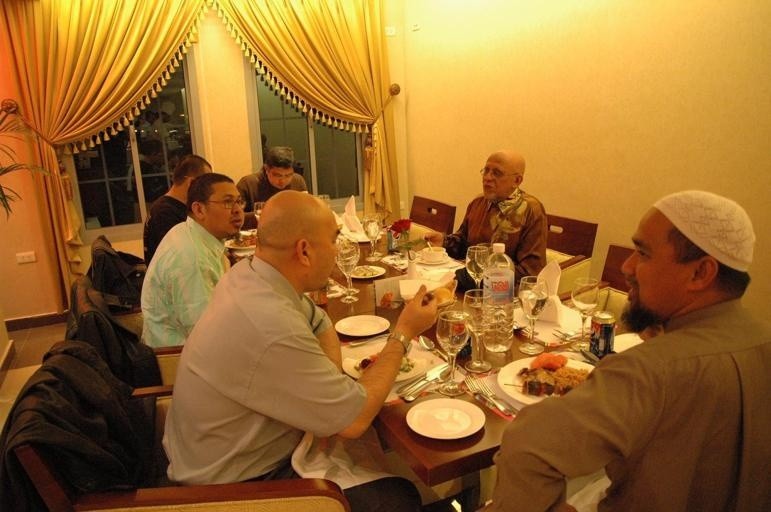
[223,190,449,306]
[336,244,643,419]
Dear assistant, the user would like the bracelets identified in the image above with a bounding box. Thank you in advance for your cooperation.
[386,332,411,354]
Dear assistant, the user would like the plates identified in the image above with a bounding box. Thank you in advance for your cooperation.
[405,397,486,440]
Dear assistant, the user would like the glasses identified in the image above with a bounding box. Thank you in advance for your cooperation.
[268,171,298,182]
[478,164,506,183]
[202,194,250,212]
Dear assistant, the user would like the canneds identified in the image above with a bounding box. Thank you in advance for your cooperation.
[387,226,400,251]
[452,323,473,359]
[589,310,616,359]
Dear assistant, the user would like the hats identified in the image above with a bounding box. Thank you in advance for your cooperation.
[648,180,760,275]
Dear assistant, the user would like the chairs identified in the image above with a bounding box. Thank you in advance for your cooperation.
[7,193,666,512]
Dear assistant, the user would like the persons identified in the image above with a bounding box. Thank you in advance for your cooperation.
[159,190,440,512]
[126,111,173,143]
[143,154,212,268]
[140,172,245,349]
[260,134,270,161]
[422,152,549,295]
[479,189,771,511]
[235,147,308,231]
